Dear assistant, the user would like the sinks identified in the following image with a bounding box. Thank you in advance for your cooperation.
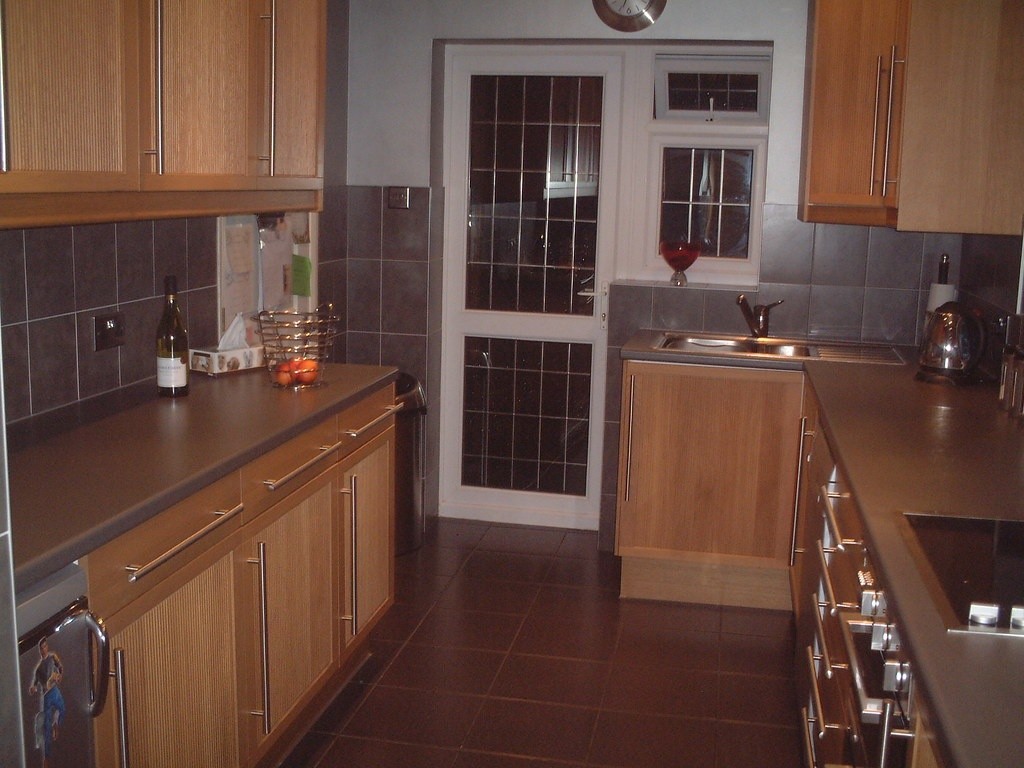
[648,329,821,359]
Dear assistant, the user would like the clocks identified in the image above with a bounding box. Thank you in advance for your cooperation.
[592,0,667,33]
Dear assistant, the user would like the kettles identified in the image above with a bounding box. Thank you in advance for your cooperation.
[913,302,985,383]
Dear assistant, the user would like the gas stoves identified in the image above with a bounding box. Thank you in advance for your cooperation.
[898,506,1024,642]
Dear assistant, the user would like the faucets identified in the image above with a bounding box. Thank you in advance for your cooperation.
[736,291,768,338]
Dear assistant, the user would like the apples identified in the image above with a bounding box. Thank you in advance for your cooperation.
[276,357,319,386]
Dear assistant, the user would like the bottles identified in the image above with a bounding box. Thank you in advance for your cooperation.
[156,276,189,399]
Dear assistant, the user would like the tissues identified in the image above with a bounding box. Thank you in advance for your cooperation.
[189,311,265,377]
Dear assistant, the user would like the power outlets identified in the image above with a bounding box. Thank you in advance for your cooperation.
[92,312,127,351]
[992,309,1011,346]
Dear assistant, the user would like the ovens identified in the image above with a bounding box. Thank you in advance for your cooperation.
[12,562,113,768]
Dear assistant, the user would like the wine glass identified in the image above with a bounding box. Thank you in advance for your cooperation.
[659,233,702,286]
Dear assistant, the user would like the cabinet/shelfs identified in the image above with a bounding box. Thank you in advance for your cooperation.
[613,359,806,614]
[77,468,250,768]
[150,0,267,220]
[268,0,334,214]
[789,370,875,768]
[240,413,340,768]
[0,0,151,230]
[339,382,395,668]
[796,0,1024,236]
